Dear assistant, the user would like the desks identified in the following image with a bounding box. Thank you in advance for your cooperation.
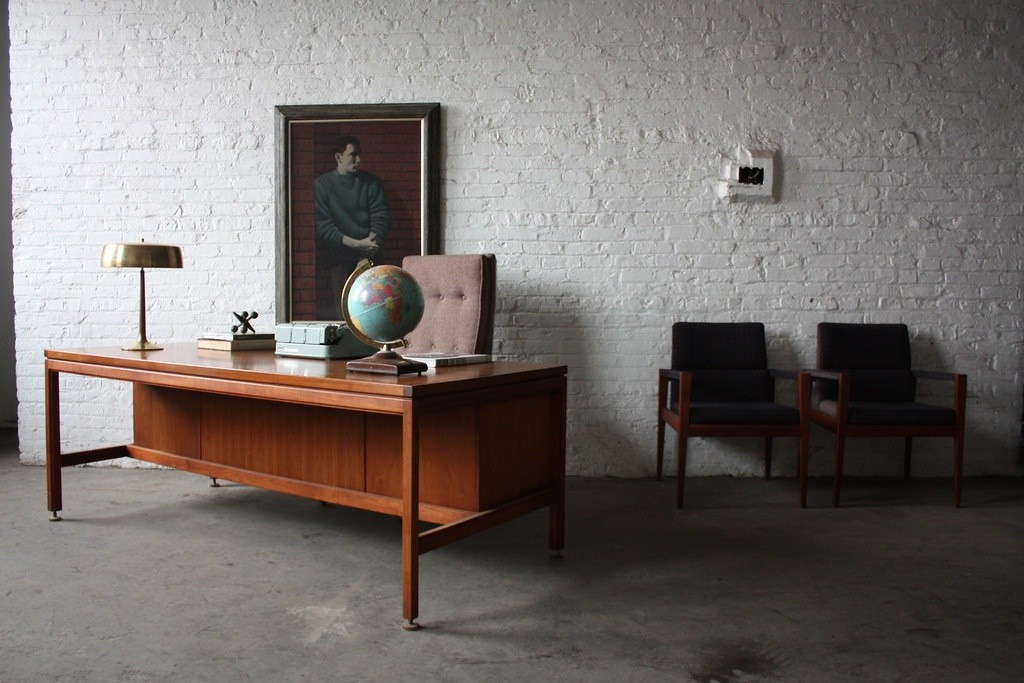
[44,342,565,631]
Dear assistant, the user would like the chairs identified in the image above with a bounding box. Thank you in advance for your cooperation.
[656,321,811,509]
[390,253,497,356]
[802,322,968,508]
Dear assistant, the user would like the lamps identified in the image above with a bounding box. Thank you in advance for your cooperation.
[101,237,183,351]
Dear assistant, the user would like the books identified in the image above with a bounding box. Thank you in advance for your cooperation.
[197,333,277,351]
[401,354,491,367]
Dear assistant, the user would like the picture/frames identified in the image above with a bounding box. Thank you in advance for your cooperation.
[274,102,442,328]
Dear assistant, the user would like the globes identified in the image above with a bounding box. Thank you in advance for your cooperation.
[340,259,429,379]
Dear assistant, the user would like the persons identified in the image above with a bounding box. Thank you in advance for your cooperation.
[314,134,391,320]
